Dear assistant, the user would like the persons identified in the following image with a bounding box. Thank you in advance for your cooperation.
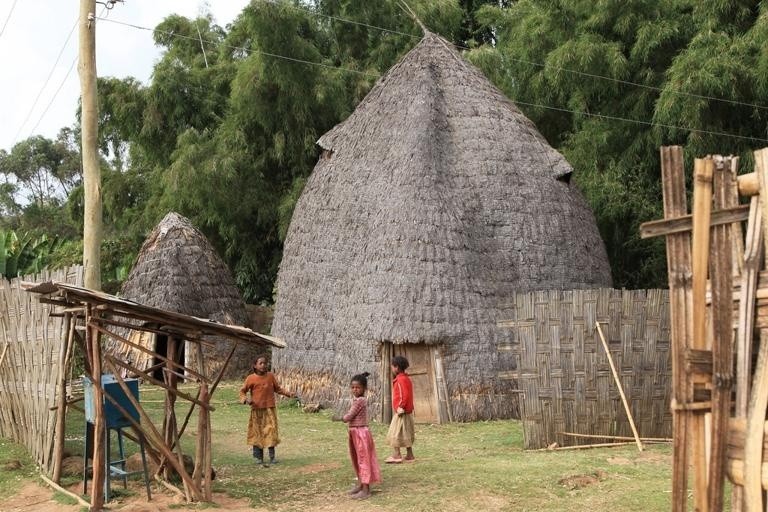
[383,354,416,465]
[237,355,298,466]
[331,372,384,502]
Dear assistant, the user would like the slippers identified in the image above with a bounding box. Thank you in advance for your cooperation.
[384,456,404,463]
[347,486,372,500]
[400,455,416,463]
